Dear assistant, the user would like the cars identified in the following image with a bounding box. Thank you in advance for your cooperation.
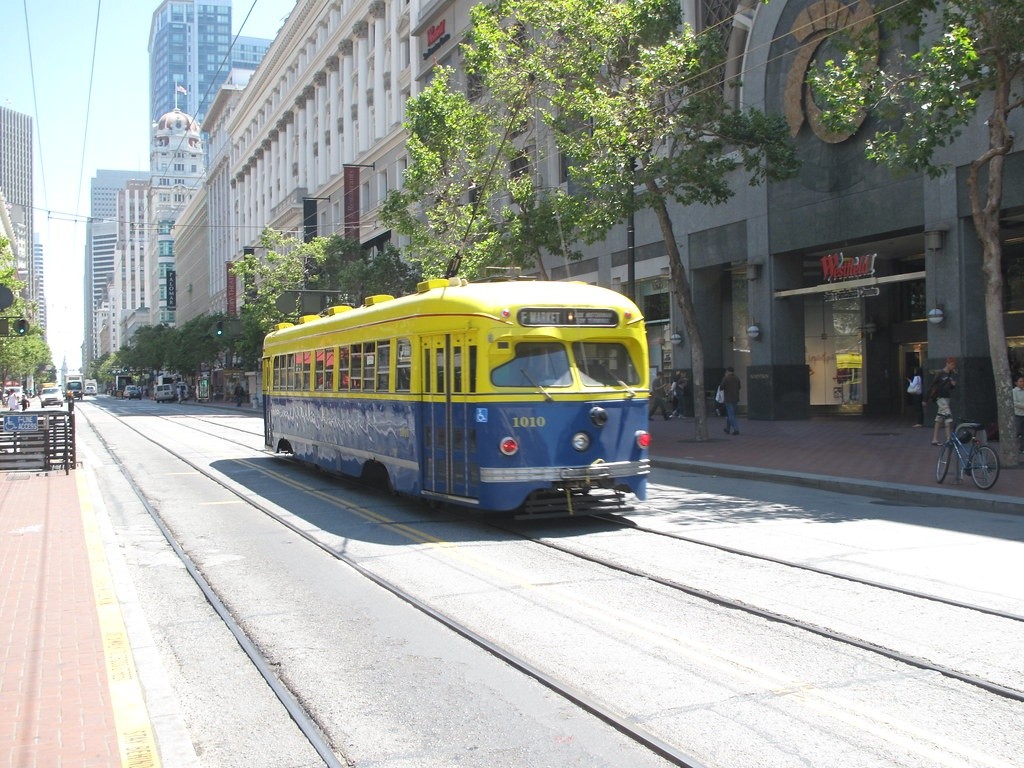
[1,371,190,408]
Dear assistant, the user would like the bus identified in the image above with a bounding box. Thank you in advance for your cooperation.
[263,276,649,524]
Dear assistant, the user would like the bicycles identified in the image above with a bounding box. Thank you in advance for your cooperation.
[933,411,1001,491]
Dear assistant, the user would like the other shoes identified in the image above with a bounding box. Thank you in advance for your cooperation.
[912,424,923,428]
[731,431,740,435]
[722,427,729,434]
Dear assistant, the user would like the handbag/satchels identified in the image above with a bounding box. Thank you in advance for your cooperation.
[715,385,725,404]
[907,385,921,394]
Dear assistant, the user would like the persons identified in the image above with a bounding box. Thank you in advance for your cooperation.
[66,391,74,414]
[668,371,688,419]
[719,367,741,436]
[921,356,958,446]
[649,372,670,421]
[1012,373,1023,464]
[234,383,244,406]
[176,386,183,404]
[8,389,18,410]
[21,395,29,411]
[904,366,924,427]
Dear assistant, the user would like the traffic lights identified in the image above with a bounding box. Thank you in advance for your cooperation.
[18,319,25,335]
